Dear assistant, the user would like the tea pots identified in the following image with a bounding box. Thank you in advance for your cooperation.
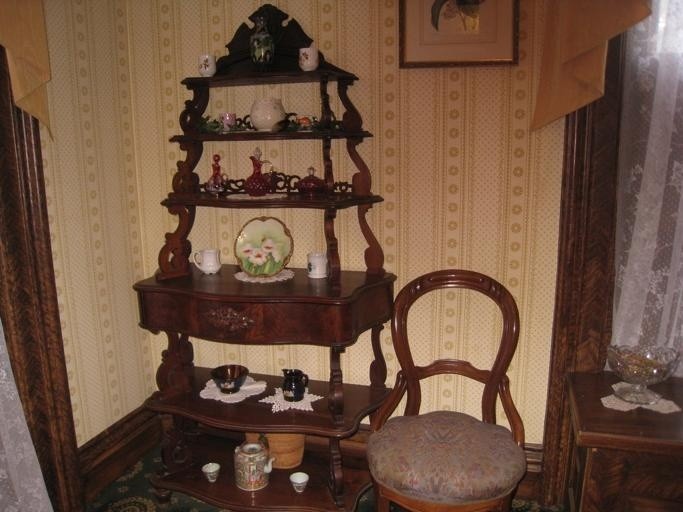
[204,154,230,197]
[231,438,275,492]
[281,368,309,403]
[245,155,275,199]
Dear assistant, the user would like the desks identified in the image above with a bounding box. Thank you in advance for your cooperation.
[562,371,683,512]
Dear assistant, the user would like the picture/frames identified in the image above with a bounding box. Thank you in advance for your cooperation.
[400,1,519,68]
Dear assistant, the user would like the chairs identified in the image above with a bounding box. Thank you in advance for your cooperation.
[367,269,527,512]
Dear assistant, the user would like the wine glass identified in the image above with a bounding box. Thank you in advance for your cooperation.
[606,343,681,406]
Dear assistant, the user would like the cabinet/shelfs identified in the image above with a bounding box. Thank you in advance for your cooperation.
[132,0,393,512]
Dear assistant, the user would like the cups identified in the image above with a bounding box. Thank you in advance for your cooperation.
[296,115,315,132]
[289,471,309,494]
[197,53,217,78]
[192,248,222,275]
[307,251,330,279]
[218,112,237,132]
[201,462,220,483]
[298,46,319,73]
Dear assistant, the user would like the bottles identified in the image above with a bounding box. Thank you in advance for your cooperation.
[250,96,286,132]
[249,15,275,75]
[295,166,328,197]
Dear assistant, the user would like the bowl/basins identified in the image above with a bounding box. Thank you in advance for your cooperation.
[209,364,249,394]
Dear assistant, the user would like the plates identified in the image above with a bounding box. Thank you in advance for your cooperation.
[233,215,294,278]
